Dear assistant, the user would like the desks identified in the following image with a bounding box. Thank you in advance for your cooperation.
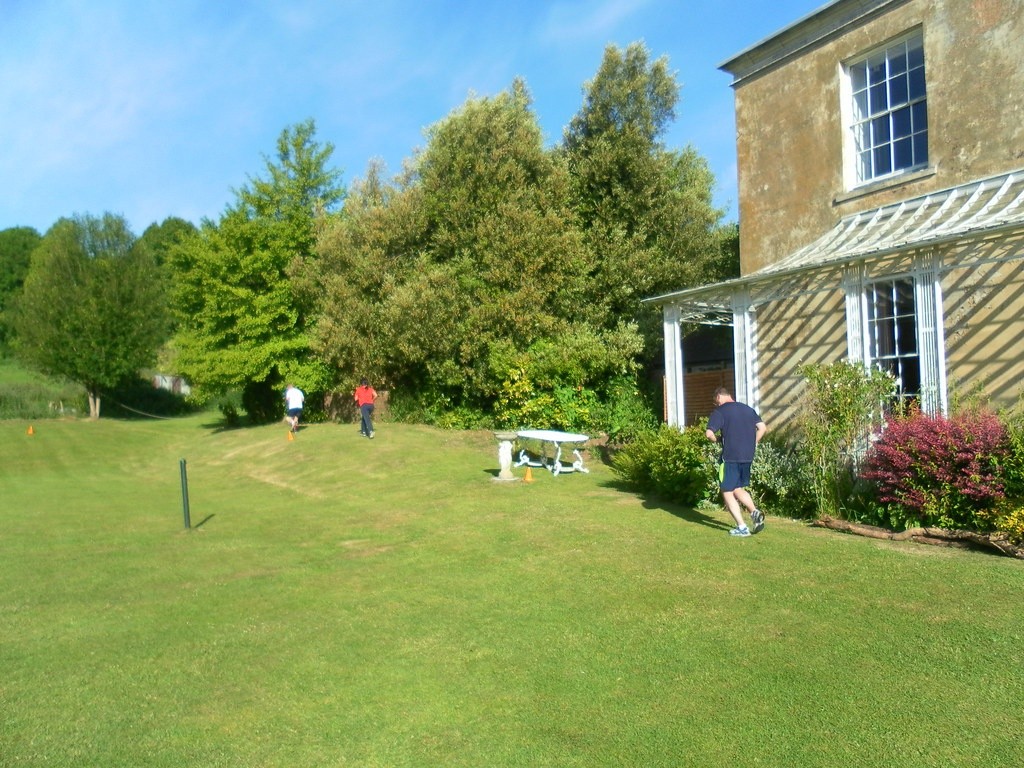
[514,430,590,476]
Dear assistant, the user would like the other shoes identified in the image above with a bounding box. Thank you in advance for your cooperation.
[362,433,366,437]
[370,432,375,439]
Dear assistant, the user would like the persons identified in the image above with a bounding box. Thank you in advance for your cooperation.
[706,386,767,537]
[354,378,378,439]
[285,384,305,432]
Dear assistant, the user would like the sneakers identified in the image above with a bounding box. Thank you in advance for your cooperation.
[750,509,765,534]
[729,525,752,536]
